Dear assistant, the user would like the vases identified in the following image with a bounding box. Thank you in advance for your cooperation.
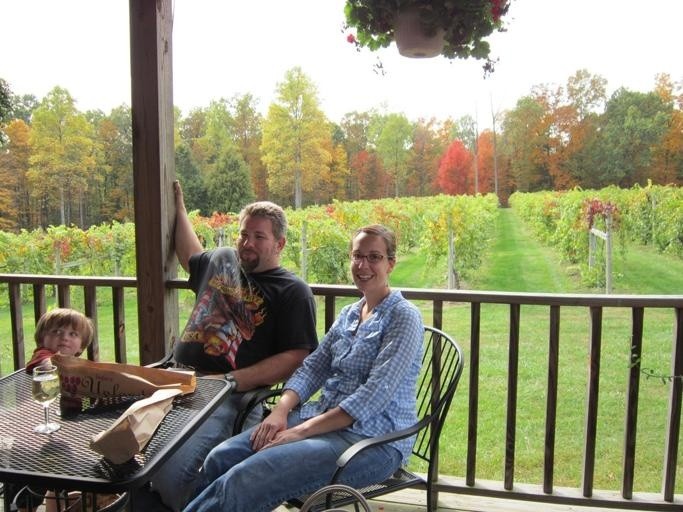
[394,2,444,58]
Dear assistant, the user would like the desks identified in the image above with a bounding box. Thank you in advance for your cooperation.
[0,367,232,511]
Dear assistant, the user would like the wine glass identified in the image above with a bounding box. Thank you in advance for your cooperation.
[30,364,62,434]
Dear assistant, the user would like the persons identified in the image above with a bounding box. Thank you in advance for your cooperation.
[180,225,426,511]
[0,306,95,510]
[137,177,320,510]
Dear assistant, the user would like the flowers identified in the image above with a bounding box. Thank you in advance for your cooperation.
[341,0,515,80]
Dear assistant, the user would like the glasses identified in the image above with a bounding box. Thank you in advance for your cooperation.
[348,252,394,264]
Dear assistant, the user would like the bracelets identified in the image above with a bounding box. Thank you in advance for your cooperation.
[222,373,236,394]
